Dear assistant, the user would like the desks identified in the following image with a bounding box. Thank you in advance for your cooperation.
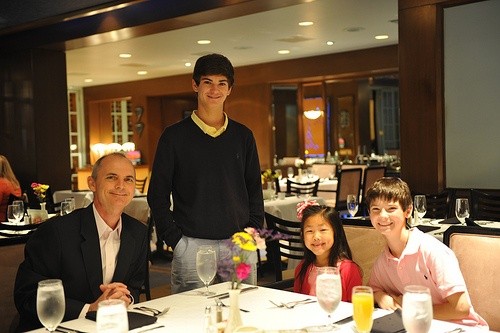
[263,193,324,223]
[54,189,150,222]
[279,175,338,207]
[351,213,500,243]
[25,281,491,333]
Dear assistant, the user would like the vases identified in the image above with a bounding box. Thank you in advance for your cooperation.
[225,288,243,333]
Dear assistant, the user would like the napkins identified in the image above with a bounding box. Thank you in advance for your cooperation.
[84,309,158,330]
[372,307,407,333]
[412,224,441,234]
[438,217,461,224]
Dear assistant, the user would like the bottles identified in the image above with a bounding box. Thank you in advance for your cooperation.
[203,306,212,333]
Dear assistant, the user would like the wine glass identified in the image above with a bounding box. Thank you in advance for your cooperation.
[414,195,428,224]
[196,250,217,309]
[315,267,342,331]
[455,198,470,226]
[346,194,358,217]
[37,279,65,333]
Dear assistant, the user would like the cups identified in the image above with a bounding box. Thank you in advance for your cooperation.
[401,286,434,333]
[7,201,24,225]
[233,326,264,333]
[278,192,285,200]
[95,299,129,333]
[352,286,374,333]
[61,198,75,216]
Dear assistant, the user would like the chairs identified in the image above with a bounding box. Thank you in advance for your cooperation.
[135,159,500,333]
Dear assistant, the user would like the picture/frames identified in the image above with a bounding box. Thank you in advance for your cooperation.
[40,203,49,220]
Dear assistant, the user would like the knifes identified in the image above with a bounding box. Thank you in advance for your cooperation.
[207,287,259,299]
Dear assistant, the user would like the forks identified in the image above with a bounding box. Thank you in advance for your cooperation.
[269,298,317,309]
[133,307,170,317]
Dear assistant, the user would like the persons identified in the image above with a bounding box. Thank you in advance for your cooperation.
[0,155,22,222]
[366,176,488,329]
[14,153,149,333]
[294,201,363,302]
[147,53,266,294]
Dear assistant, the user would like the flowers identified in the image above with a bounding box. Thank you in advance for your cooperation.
[296,197,319,220]
[230,227,267,289]
[32,182,49,203]
